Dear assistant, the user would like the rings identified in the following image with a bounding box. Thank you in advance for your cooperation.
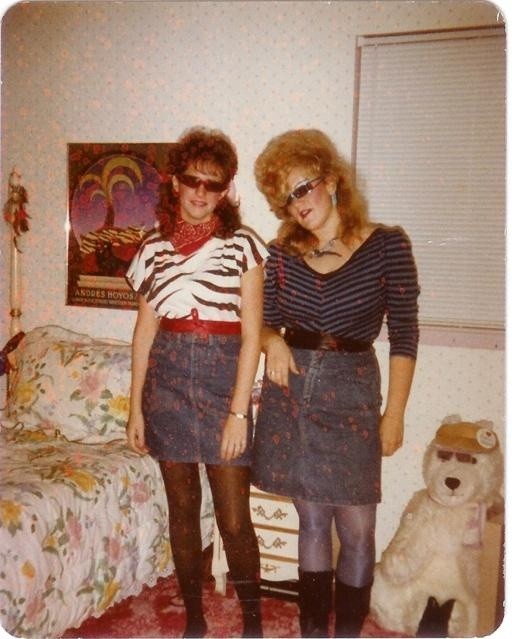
[270,370,275,375]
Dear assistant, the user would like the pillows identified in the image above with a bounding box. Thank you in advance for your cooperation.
[7,322,132,449]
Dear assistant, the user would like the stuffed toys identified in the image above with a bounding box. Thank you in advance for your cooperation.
[367,415,506,638]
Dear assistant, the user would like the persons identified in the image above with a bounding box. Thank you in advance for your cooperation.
[250,130,420,637]
[124,123,271,638]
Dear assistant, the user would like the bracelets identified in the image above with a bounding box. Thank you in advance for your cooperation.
[227,409,250,421]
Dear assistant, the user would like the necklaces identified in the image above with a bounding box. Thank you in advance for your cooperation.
[306,233,343,261]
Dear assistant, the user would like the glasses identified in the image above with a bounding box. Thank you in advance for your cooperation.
[176,174,226,193]
[287,178,323,204]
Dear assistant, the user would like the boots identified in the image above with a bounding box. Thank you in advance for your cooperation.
[335,578,371,638]
[297,568,333,637]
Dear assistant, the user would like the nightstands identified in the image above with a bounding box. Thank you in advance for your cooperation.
[209,470,341,603]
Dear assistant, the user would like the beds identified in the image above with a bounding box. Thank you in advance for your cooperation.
[0,366,213,638]
